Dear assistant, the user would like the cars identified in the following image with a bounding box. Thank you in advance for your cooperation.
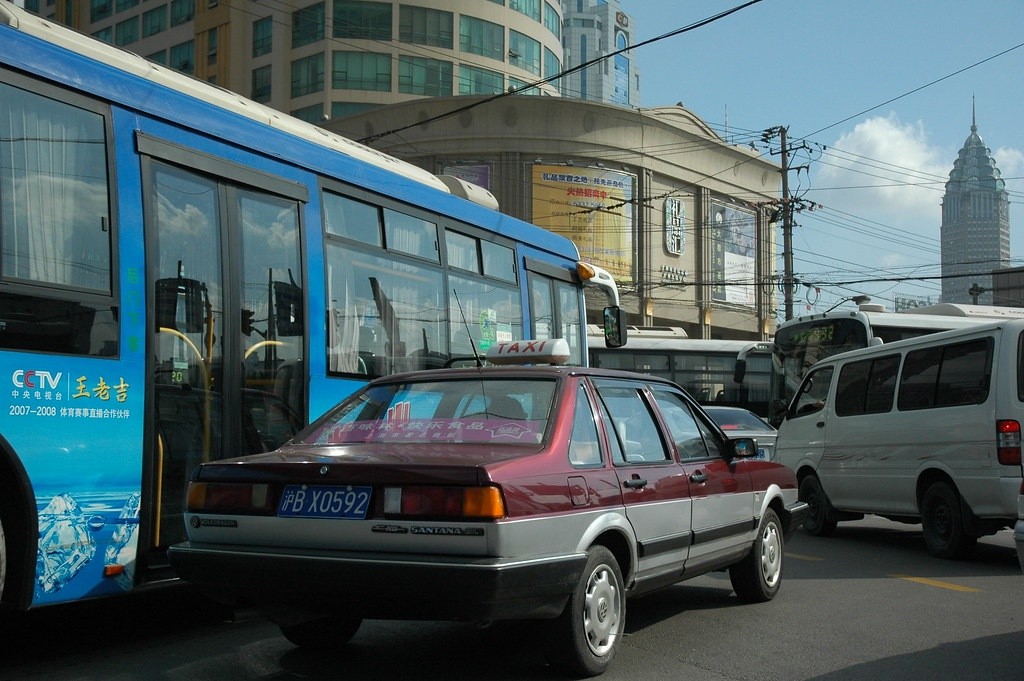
[656,406,782,464]
[166,336,811,678]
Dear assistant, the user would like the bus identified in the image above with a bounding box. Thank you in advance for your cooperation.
[731,302,1024,435]
[0,1,631,650]
[462,337,780,432]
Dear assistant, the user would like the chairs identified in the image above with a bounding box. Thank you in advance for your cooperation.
[699,388,711,401]
[716,390,725,402]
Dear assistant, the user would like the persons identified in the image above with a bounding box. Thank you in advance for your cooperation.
[482,396,529,420]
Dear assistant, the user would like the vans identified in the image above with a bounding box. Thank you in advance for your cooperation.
[768,319,1024,561]
